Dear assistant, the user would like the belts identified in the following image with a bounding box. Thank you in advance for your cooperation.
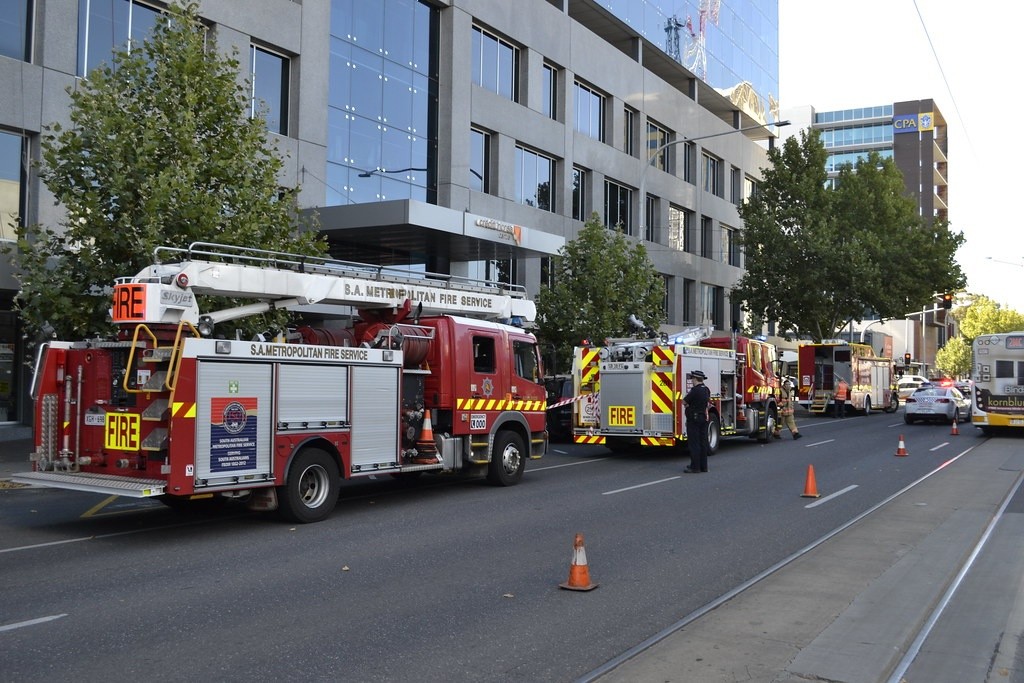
[689,413,706,416]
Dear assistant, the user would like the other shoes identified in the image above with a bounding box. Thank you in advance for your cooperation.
[793,433,802,440]
[686,465,691,469]
[683,469,701,473]
[775,435,781,439]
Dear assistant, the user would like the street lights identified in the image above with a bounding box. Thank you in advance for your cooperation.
[637,120,793,245]
[356,165,482,183]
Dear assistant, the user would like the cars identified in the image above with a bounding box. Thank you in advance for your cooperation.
[902,378,971,426]
[896,374,971,399]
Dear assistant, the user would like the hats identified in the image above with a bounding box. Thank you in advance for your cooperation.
[689,370,708,380]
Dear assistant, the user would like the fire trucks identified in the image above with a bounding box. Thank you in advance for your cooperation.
[570,324,789,457]
[795,339,900,417]
[0,240,561,526]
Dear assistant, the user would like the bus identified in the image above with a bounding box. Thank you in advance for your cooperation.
[970,330,1024,435]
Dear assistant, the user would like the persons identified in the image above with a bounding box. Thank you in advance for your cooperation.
[682,370,711,473]
[773,380,803,440]
[833,377,848,419]
[793,373,798,402]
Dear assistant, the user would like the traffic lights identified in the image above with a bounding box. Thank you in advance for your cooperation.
[905,353,911,364]
[942,293,952,310]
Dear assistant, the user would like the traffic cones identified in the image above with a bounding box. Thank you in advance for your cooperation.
[950,420,960,435]
[799,464,822,498]
[558,533,600,591]
[895,434,909,456]
